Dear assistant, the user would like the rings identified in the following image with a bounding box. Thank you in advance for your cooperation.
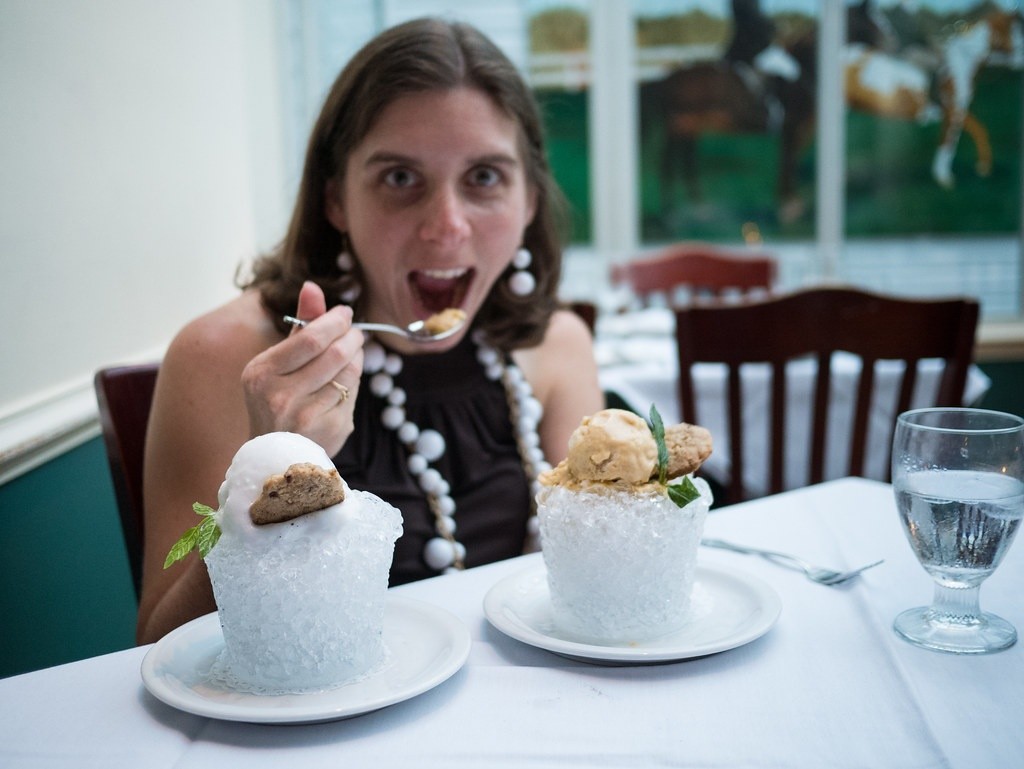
[332,378,349,407]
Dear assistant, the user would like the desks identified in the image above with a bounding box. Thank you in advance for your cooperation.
[0,474,1024,767]
[593,307,993,502]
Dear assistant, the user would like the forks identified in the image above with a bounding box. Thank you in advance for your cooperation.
[702,537,882,585]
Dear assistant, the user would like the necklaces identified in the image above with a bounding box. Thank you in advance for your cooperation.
[363,330,553,575]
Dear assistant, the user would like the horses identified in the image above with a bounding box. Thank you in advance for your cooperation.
[639,16,818,231]
[843,0,1024,194]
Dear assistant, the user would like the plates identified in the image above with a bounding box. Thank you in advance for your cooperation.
[484,559,785,665]
[139,591,485,723]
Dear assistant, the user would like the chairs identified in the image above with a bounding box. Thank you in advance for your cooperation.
[94,355,172,600]
[615,251,777,313]
[675,283,981,507]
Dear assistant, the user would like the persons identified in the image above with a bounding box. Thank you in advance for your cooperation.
[136,17,603,645]
[729,1,774,99]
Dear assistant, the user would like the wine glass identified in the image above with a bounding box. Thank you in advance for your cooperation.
[890,407,1024,655]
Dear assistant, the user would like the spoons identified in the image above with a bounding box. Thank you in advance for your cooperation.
[284,314,463,343]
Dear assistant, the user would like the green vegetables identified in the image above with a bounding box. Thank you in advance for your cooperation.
[649,403,701,509]
[162,500,223,570]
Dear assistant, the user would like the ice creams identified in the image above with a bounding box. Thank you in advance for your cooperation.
[537,404,714,500]
[207,429,352,547]
[423,308,468,335]
